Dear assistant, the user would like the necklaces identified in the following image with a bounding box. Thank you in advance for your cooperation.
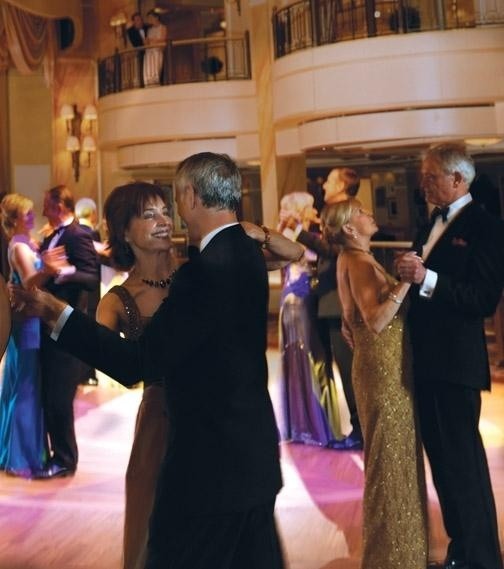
[132,266,178,290]
[340,246,376,257]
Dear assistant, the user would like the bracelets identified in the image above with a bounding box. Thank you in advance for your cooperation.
[260,225,273,246]
[388,293,403,305]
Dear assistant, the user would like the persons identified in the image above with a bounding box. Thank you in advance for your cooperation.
[142,10,169,87]
[320,199,429,568]
[338,139,504,567]
[277,170,362,449]
[1,182,114,480]
[126,12,164,87]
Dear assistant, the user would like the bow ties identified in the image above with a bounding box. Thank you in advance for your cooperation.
[187,245,199,259]
[431,207,449,223]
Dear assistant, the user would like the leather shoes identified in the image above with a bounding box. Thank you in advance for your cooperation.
[36,462,75,479]
[327,437,362,450]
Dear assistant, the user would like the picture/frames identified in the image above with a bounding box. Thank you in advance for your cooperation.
[387,197,400,220]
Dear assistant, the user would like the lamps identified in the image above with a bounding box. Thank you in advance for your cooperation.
[60,103,98,183]
